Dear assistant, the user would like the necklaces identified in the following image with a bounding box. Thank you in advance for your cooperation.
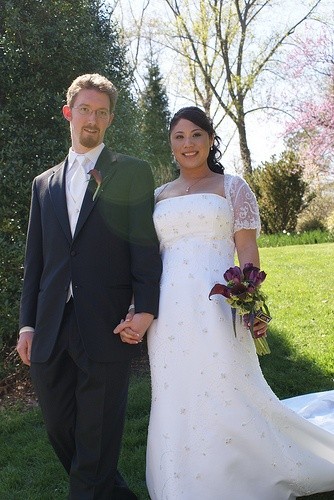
[182,177,201,192]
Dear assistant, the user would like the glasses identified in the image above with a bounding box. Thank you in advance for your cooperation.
[70,103,111,120]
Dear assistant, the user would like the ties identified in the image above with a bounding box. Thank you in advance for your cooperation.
[70,155,88,203]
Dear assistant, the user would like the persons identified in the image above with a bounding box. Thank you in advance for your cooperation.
[14,73,164,500]
[119,107,334,500]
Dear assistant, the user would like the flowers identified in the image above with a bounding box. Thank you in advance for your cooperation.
[209,263,272,356]
[85,170,102,202]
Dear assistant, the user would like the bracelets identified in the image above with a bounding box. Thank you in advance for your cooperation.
[127,304,135,312]
